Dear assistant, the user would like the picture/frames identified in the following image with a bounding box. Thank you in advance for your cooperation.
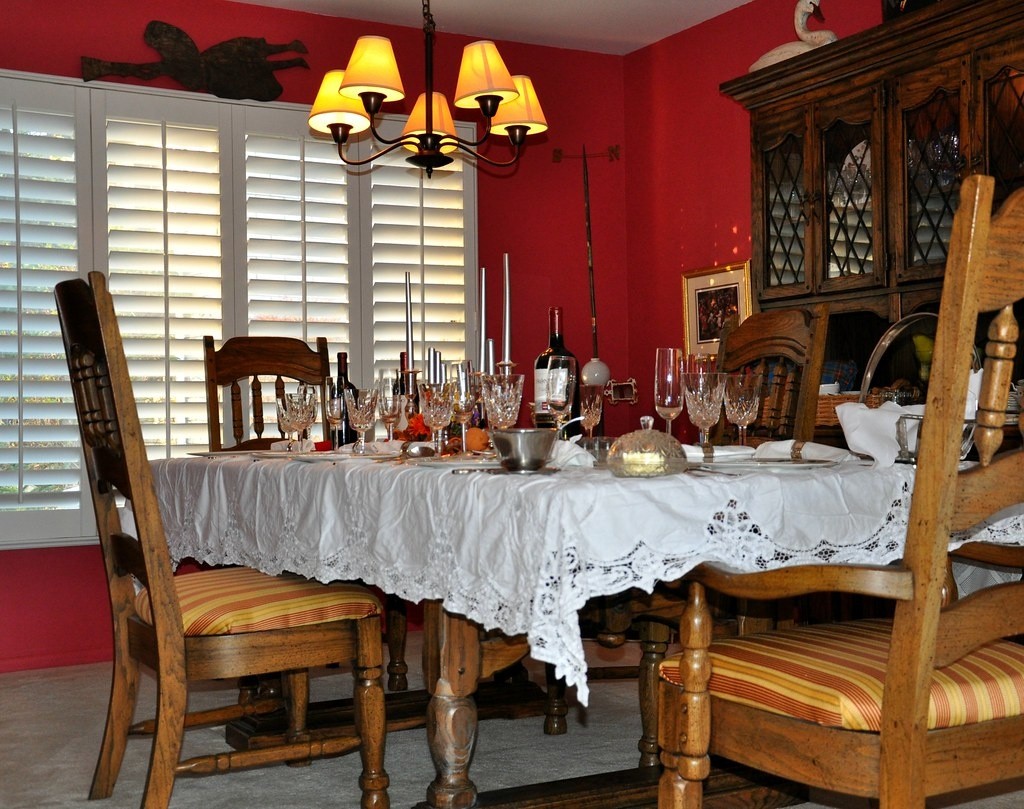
[680,258,754,362]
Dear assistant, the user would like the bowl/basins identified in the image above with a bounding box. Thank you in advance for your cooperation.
[485,428,558,473]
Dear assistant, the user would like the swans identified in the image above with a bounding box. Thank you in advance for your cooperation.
[748,0,838,73]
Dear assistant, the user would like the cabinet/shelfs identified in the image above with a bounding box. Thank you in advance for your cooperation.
[718,0,1024,449]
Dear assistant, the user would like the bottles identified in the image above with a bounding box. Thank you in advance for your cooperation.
[534,306,579,440]
[394,352,419,433]
[331,352,357,450]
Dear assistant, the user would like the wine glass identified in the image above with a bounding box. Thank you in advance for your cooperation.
[579,384,603,437]
[654,349,763,447]
[277,361,525,452]
[545,355,575,438]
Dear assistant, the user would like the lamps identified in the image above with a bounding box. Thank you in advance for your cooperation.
[308,0,549,180]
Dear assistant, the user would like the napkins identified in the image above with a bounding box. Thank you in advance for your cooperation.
[835,367,984,467]
[682,441,755,464]
[546,434,597,469]
[271,440,316,453]
[338,440,437,453]
[753,439,861,463]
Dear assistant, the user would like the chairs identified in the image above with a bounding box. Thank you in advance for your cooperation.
[543,299,832,767]
[54,270,390,809]
[656,175,1024,809]
[202,336,409,702]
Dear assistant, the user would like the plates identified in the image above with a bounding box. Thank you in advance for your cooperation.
[1005,390,1020,424]
[188,450,557,471]
[702,463,839,473]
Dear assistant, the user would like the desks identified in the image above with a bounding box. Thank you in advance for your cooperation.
[116,436,1024,809]
